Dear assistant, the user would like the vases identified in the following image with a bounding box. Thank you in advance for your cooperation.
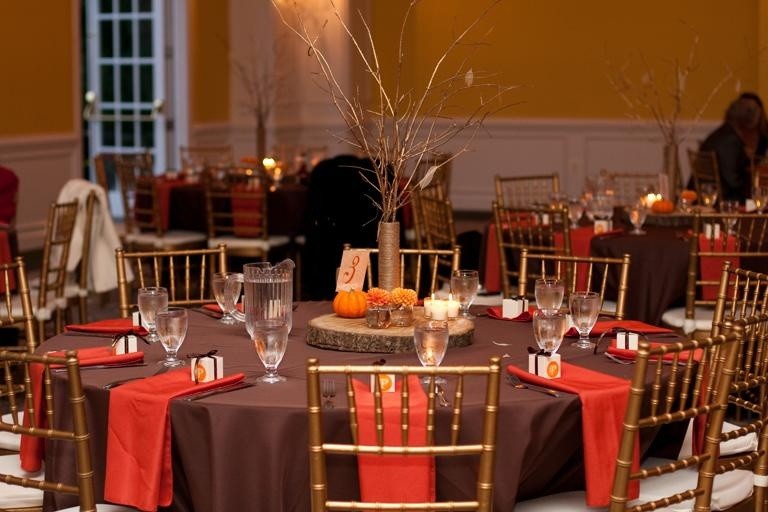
[255,113,267,162]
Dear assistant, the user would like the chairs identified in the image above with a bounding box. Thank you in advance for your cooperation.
[709,261,768,428]
[115,244,227,318]
[0,256,36,453]
[94,150,156,244]
[415,165,484,292]
[662,208,768,341]
[28,189,95,324]
[0,350,144,512]
[411,153,485,290]
[202,163,306,301]
[518,248,630,321]
[176,144,234,175]
[606,173,661,209]
[304,357,501,512]
[640,312,768,512]
[492,200,570,307]
[119,154,207,293]
[512,324,744,512]
[0,197,79,344]
[686,149,723,208]
[343,244,461,299]
[495,174,561,208]
[273,137,330,174]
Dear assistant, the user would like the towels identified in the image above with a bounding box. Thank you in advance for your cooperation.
[608,338,720,459]
[230,185,263,237]
[554,226,623,294]
[563,321,672,337]
[507,360,639,507]
[202,303,245,315]
[400,177,425,241]
[19,346,144,472]
[496,213,537,221]
[484,220,556,292]
[348,375,436,504]
[151,179,190,231]
[487,306,568,322]
[104,367,244,511]
[690,231,740,303]
[63,318,149,337]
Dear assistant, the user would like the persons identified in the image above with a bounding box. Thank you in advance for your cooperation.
[686,89,768,210]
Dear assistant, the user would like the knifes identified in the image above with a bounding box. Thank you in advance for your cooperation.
[56,364,105,372]
[187,382,253,403]
[192,307,213,317]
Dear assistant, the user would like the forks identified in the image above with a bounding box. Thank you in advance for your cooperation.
[476,313,489,317]
[63,331,116,340]
[103,367,169,389]
[506,373,561,399]
[322,378,336,409]
[604,350,692,372]
[614,330,679,339]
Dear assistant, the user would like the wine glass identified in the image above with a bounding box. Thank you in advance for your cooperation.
[751,185,768,214]
[534,309,566,354]
[570,291,601,351]
[155,306,189,367]
[211,273,243,326]
[450,269,479,321]
[720,201,740,236]
[568,201,583,229]
[629,208,648,236]
[225,258,295,341]
[700,182,720,207]
[254,319,289,385]
[592,196,614,220]
[414,318,449,385]
[137,287,169,343]
[535,278,564,317]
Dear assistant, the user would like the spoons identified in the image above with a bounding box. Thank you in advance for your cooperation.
[434,384,452,408]
[293,303,299,311]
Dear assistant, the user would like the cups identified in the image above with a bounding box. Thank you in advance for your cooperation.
[389,306,413,327]
[366,309,389,329]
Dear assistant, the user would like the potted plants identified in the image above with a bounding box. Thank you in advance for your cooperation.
[606,21,768,202]
[270,0,524,291]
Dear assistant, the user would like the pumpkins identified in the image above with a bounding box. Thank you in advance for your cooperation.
[653,200,674,213]
[333,287,366,317]
